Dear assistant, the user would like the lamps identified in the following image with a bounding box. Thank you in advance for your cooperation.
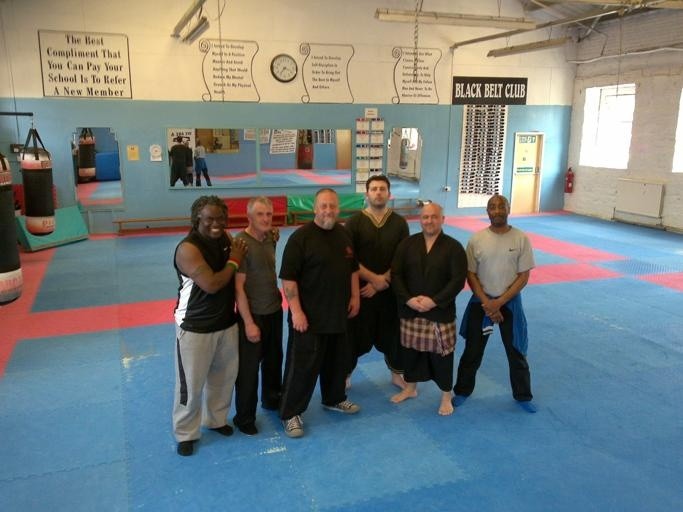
[376,0,537,30]
[488,26,573,57]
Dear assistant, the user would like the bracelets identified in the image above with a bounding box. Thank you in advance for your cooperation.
[225,258,241,272]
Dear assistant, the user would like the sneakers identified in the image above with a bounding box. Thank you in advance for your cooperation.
[321,401,358,414]
[283,414,304,436]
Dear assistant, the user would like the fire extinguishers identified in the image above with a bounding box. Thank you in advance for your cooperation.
[564,168,574,193]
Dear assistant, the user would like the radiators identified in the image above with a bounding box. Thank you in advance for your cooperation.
[612,179,666,231]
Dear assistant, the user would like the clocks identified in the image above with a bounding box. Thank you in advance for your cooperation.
[270,54,298,83]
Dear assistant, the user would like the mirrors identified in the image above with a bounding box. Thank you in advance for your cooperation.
[258,128,352,189]
[70,127,126,211]
[386,127,423,200]
[164,127,256,190]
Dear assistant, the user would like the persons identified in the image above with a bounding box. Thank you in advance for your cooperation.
[234,195,281,433]
[452,194,538,413]
[168,136,212,187]
[173,194,280,458]
[390,204,468,416]
[344,176,409,390]
[278,189,362,437]
